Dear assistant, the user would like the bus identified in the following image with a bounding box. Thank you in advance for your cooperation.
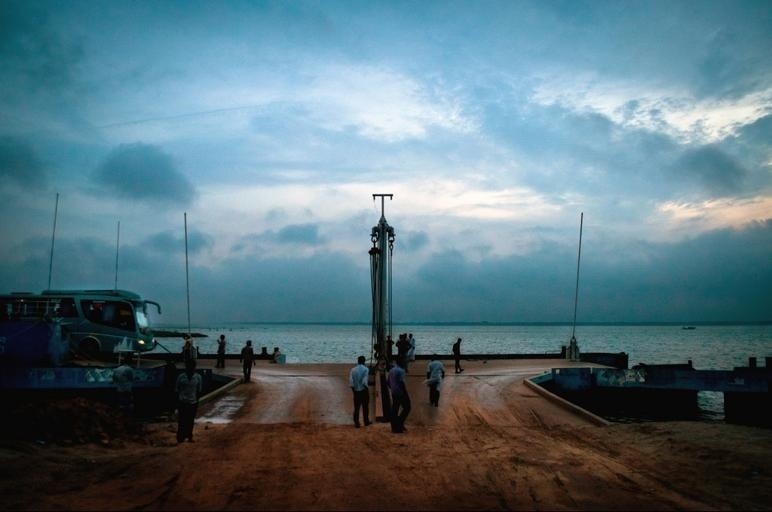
[1,289,162,362]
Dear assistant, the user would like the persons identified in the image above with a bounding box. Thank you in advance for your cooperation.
[112,359,136,418]
[215,335,226,368]
[240,340,255,383]
[183,334,192,363]
[175,359,203,442]
[349,356,372,428]
[427,354,444,407]
[453,338,464,373]
[387,333,415,431]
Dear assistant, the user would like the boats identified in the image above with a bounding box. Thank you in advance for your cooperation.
[682,326,697,330]
[0,317,213,409]
